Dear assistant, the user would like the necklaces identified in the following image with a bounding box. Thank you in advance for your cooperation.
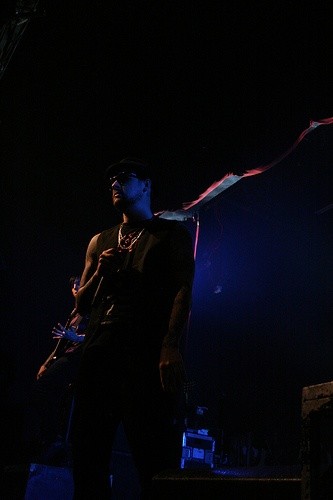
[114,221,150,253]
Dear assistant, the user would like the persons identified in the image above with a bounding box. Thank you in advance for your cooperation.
[37,272,84,379]
[74,156,198,500]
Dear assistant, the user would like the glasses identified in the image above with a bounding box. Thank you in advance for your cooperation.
[106,174,146,192]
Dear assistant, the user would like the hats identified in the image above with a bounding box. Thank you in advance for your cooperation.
[111,155,153,178]
